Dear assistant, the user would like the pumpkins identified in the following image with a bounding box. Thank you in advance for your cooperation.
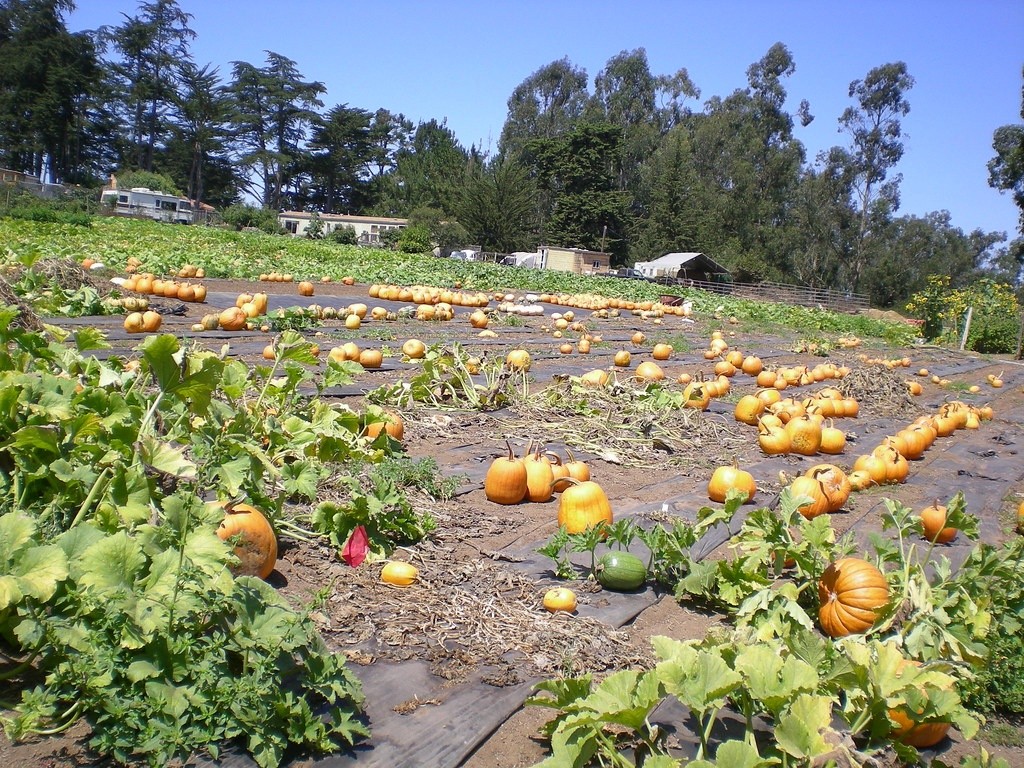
[82,257,1024,746]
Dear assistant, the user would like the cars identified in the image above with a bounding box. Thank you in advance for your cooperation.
[450,250,479,262]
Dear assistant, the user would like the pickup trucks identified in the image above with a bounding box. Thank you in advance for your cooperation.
[596,268,656,283]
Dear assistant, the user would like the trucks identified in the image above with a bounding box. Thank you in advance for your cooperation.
[100,188,193,224]
